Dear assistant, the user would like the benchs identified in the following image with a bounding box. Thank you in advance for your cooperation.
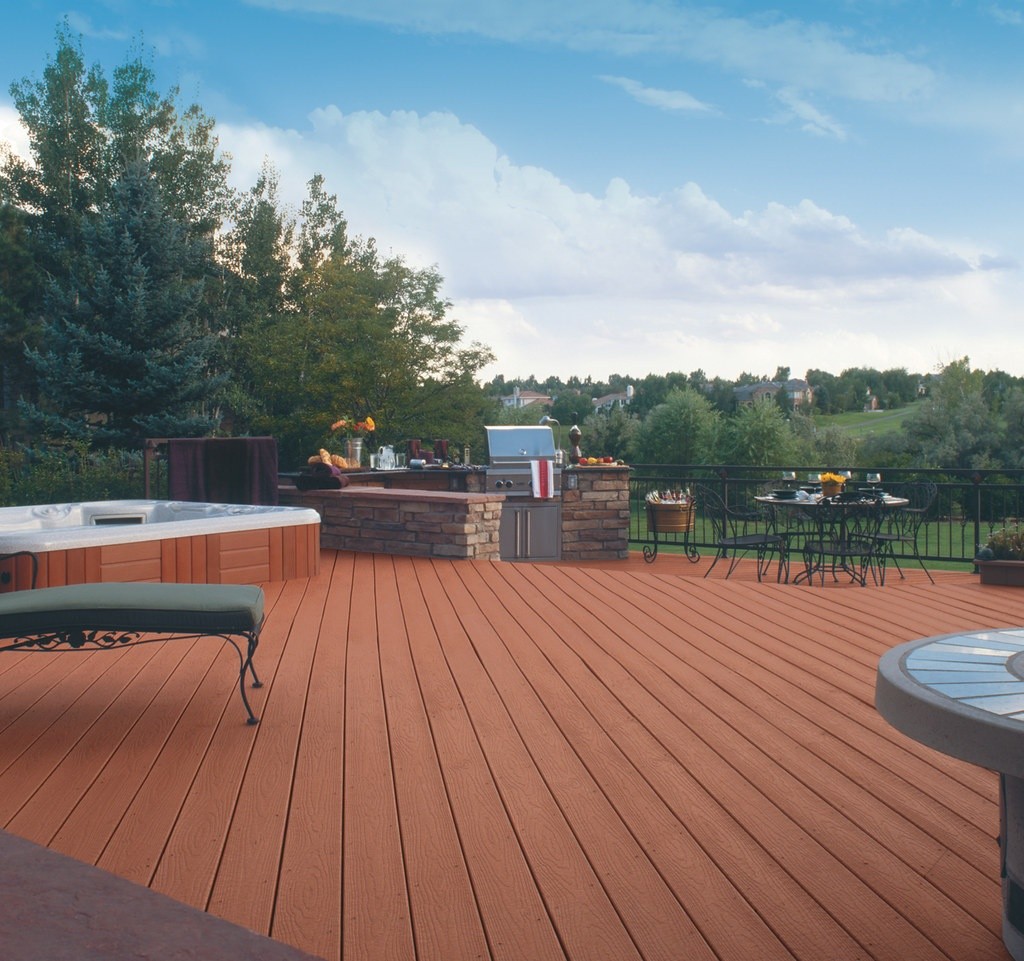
[0,552,265,724]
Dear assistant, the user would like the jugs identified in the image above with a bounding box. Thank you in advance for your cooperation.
[378,445,395,470]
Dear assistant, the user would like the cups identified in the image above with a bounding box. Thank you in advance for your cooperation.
[369,453,379,470]
[396,453,405,468]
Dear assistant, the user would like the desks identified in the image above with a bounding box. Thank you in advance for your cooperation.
[875,628,1024,961]
[754,495,909,587]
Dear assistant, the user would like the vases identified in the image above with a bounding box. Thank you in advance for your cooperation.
[344,437,363,468]
[822,482,842,496]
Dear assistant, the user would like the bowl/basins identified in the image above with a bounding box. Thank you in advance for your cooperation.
[858,488,882,494]
[773,489,796,496]
[800,487,822,495]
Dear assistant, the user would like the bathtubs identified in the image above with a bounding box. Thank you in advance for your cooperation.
[0,498,322,595]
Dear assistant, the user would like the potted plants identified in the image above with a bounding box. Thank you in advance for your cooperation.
[973,521,1024,587]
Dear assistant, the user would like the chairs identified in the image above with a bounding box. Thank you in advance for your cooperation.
[696,478,938,587]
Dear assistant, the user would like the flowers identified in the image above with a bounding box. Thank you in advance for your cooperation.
[331,415,376,438]
[820,471,846,482]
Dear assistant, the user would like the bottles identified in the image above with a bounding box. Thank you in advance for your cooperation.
[659,488,690,504]
[464,443,471,465]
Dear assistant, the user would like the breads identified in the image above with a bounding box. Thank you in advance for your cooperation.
[308,448,349,468]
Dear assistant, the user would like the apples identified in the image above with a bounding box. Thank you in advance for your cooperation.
[580,456,613,464]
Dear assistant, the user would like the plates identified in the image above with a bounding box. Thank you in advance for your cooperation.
[772,495,798,499]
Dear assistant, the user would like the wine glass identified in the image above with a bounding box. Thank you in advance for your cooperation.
[808,474,822,493]
[838,471,852,493]
[782,471,796,489]
[866,473,881,495]
